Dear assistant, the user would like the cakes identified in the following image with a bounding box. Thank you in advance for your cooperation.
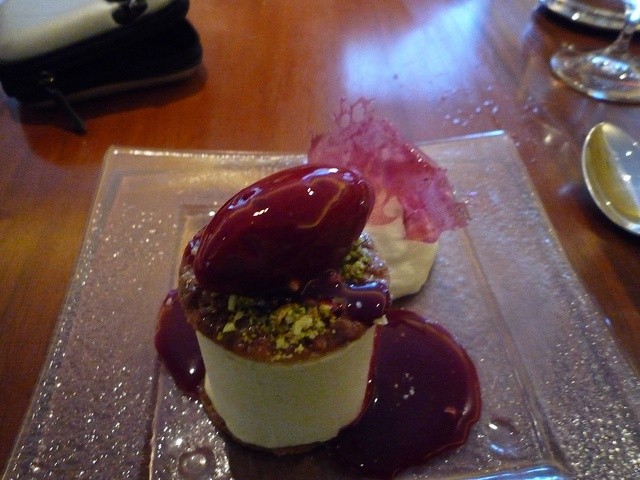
[176,164,392,448]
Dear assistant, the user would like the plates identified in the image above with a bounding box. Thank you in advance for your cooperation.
[0,128,640,480]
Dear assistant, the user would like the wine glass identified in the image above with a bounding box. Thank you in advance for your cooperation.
[551,10,640,104]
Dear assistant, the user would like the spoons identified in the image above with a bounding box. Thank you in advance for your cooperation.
[583,120,639,237]
